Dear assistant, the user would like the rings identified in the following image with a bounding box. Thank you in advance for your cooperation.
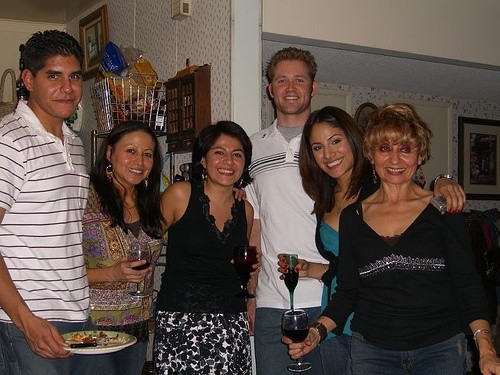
[258,267,261,272]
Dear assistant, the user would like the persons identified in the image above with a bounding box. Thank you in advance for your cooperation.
[0,29,90,375]
[82,46,500,375]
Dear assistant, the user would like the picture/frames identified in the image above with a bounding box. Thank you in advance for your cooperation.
[457,116,500,201]
[79,4,109,81]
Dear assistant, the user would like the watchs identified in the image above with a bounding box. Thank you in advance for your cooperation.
[434,173,456,184]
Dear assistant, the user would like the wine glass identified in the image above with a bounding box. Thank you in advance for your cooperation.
[128,242,152,298]
[283,254,299,310]
[281,310,312,372]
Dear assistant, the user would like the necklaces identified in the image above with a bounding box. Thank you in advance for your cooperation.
[335,190,348,213]
[124,204,137,209]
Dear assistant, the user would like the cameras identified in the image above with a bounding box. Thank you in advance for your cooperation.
[430,196,447,212]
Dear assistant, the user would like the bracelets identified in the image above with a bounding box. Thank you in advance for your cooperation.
[318,263,328,282]
[246,293,255,298]
[473,328,495,349]
[309,321,327,344]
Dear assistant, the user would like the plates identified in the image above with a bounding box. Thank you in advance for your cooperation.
[57,331,137,354]
